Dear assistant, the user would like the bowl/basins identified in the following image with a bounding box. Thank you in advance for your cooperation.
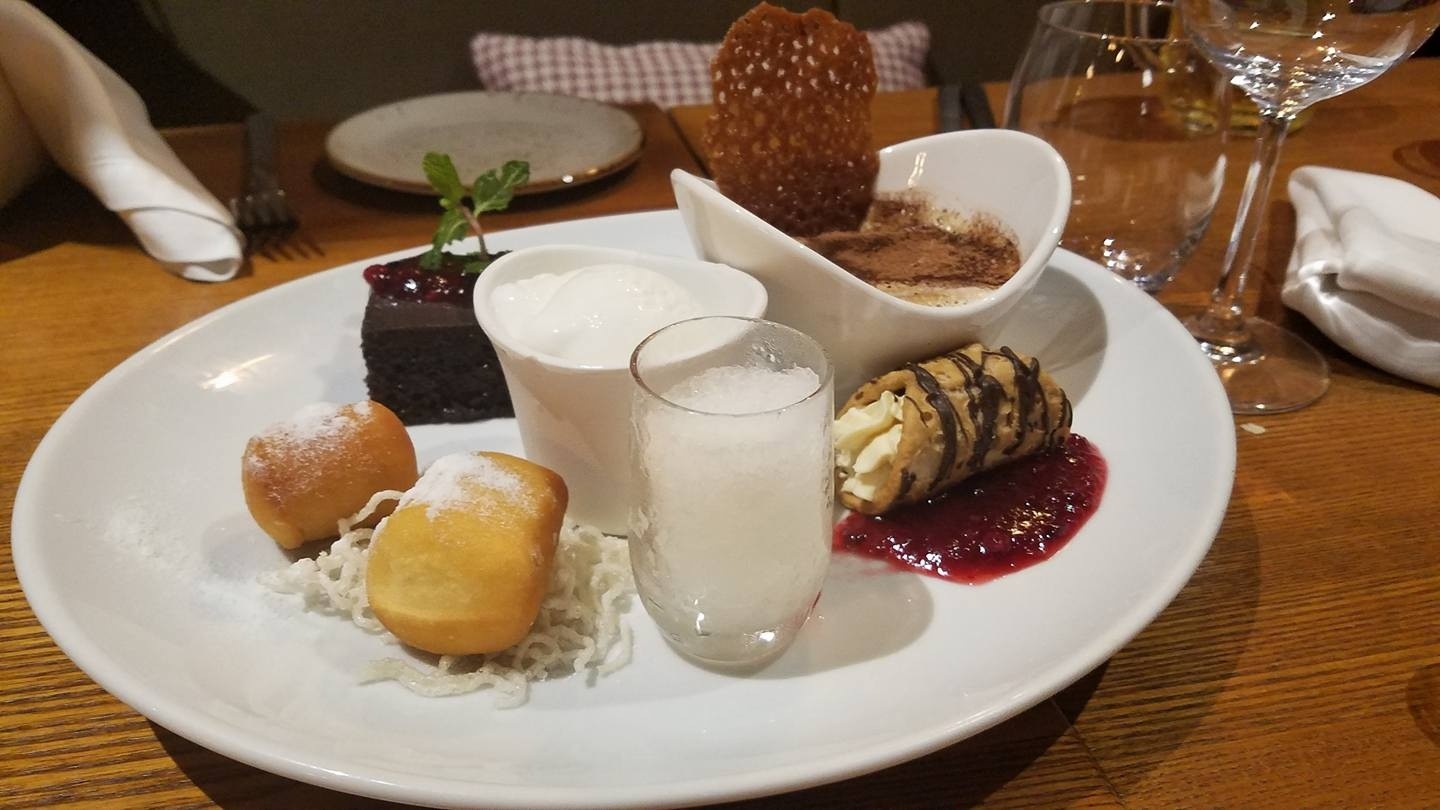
[671,129,1073,417]
[471,244,767,537]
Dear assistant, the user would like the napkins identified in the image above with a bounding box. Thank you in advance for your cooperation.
[2,3,245,285]
[1283,165,1439,388]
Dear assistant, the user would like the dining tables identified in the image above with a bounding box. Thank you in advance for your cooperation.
[1,66,1438,810]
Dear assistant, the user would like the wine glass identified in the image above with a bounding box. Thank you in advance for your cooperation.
[1167,1,1438,417]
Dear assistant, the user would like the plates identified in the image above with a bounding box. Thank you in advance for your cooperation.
[11,210,1237,810]
[320,90,646,195]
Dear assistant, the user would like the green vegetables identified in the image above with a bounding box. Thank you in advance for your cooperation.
[420,154,526,254]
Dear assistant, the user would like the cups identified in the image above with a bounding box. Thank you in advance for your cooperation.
[997,0,1228,293]
[625,315,834,663]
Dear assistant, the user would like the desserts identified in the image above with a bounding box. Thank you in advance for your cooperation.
[365,444,572,648]
[347,244,520,425]
[233,396,425,551]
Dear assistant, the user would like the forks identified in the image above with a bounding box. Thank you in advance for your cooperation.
[227,115,306,243]
